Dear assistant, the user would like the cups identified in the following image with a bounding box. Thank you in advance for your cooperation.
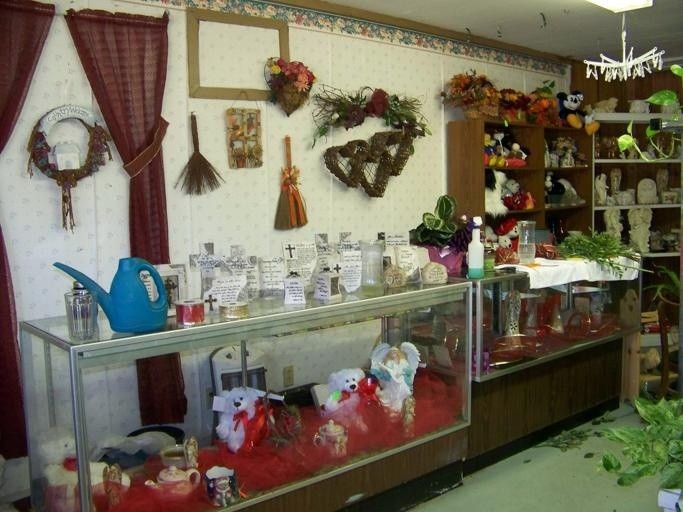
[516,220,538,263]
[205,464,241,508]
[159,444,186,469]
[358,239,387,297]
[64,292,100,341]
[627,99,651,113]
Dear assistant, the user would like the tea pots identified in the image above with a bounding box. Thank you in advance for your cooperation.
[144,466,202,508]
[313,419,350,460]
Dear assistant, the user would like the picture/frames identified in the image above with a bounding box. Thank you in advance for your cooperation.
[185,6,291,102]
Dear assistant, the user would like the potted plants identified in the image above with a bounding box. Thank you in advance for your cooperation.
[408,193,464,273]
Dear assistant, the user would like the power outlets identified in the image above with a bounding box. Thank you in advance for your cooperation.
[206,389,216,409]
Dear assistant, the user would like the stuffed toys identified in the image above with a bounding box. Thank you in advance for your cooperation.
[36,426,130,511]
[213,388,258,452]
[544,133,591,247]
[556,89,599,138]
[328,367,367,433]
[486,125,537,265]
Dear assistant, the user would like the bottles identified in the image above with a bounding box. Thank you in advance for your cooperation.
[484,246,496,272]
[376,231,396,268]
[73,281,98,337]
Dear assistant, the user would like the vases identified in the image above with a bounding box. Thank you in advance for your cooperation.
[463,108,483,120]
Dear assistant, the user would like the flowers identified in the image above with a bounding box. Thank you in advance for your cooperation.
[312,84,432,151]
[498,88,531,120]
[265,57,317,106]
[441,73,502,108]
[531,79,563,126]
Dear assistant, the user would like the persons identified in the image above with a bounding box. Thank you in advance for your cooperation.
[369,342,420,422]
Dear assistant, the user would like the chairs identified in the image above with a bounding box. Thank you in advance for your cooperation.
[657,300,682,399]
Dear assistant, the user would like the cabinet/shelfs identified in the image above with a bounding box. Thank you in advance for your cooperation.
[593,113,683,257]
[19,279,473,512]
[447,121,593,244]
[472,253,640,467]
